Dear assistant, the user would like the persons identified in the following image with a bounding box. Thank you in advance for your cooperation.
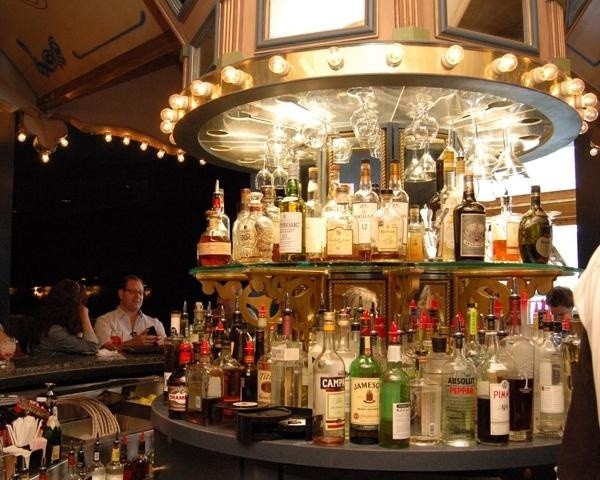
[554,242,600,479]
[545,286,574,323]
[24,278,101,360]
[93,274,167,356]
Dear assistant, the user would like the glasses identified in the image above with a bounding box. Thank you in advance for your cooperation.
[122,289,143,296]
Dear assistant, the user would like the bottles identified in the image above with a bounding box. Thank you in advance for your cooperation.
[198,157,552,266]
[34,378,152,480]
[168,293,571,444]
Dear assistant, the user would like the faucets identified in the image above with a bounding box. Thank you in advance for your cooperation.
[35,382,56,406]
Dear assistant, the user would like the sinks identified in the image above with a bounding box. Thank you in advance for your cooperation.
[58,400,153,441]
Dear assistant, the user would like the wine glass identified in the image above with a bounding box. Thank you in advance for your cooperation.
[110,330,125,352]
[255,82,534,188]
